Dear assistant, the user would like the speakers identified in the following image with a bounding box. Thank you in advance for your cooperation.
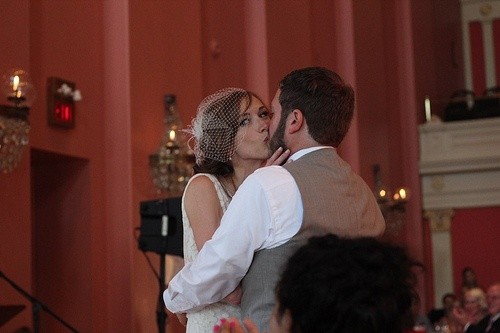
[138,196,184,259]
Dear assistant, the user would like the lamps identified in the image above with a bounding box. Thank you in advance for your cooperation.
[0,70,38,117]
[377,183,411,214]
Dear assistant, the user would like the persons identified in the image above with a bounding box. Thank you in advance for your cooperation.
[465,283,500,333]
[213,234,427,333]
[182,87,291,333]
[164,67,386,333]
[410,261,488,333]
